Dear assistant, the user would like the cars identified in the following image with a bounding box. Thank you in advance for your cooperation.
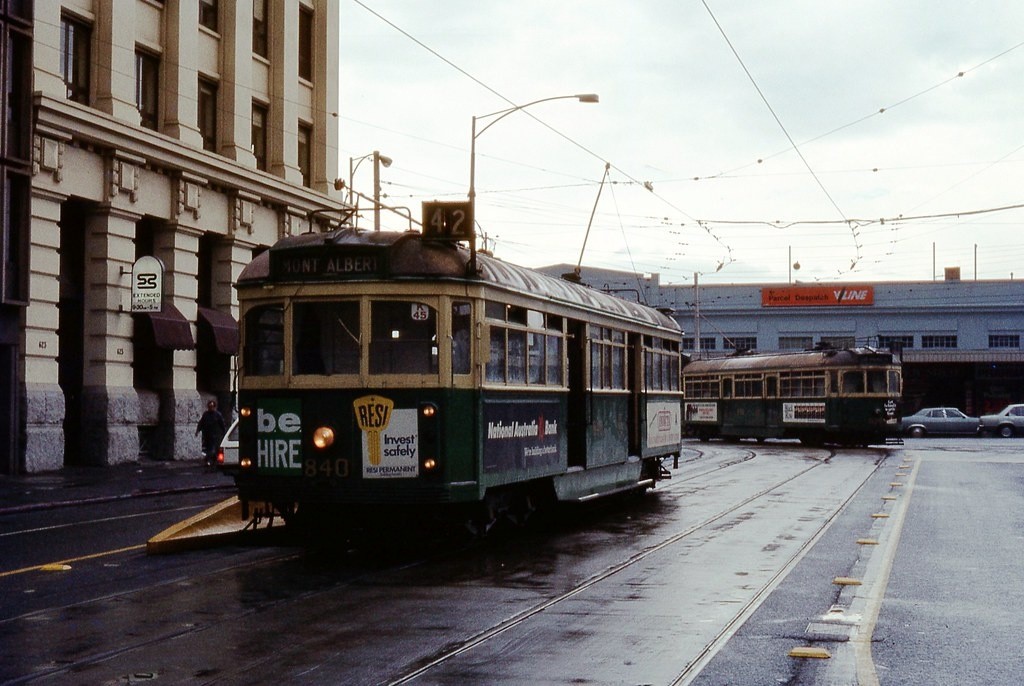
[218,416,239,475]
[902,406,982,438]
[980,404,1024,438]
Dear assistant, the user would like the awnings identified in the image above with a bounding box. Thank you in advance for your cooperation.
[147,301,196,352]
[200,308,239,356]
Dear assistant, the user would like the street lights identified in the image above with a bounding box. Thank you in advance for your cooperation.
[468,93,599,201]
[335,151,392,231]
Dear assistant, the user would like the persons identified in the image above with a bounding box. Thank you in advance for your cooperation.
[194,400,227,472]
[247,332,284,375]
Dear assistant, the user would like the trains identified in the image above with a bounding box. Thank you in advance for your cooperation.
[683,335,904,448]
[232,201,683,537]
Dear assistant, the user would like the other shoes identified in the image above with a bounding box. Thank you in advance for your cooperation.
[211,466,216,473]
[204,466,208,473]
